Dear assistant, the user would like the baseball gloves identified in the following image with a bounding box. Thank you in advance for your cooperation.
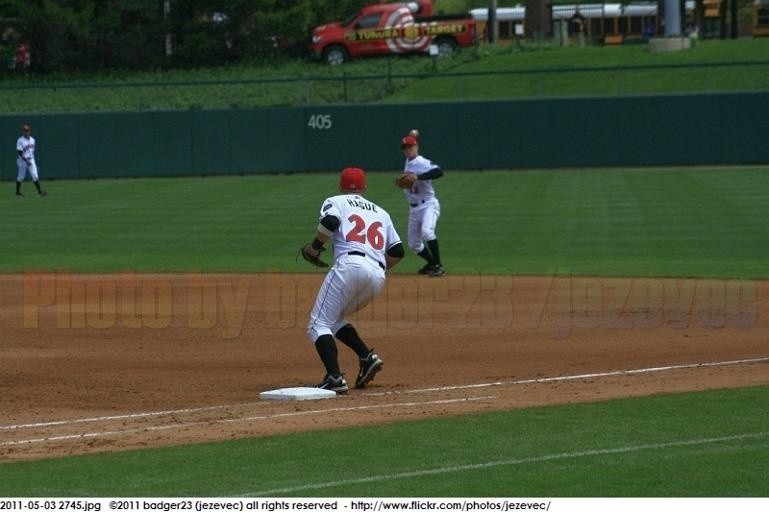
[300,244,329,268]
[395,172,416,190]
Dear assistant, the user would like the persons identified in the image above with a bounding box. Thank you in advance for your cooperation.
[300,166,405,395]
[15,123,48,200]
[395,130,446,279]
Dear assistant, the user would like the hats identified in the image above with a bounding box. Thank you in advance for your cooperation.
[401,136,417,148]
[339,167,365,192]
[23,124,30,131]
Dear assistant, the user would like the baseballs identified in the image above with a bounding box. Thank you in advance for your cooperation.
[409,130,417,136]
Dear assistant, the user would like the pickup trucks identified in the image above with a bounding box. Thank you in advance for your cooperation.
[310,2,477,66]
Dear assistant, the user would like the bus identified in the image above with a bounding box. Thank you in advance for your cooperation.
[469,1,736,53]
[750,1,769,39]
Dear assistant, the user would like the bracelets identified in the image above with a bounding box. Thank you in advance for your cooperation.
[311,237,325,251]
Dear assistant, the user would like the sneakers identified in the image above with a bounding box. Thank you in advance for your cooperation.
[310,374,349,392]
[16,191,22,196]
[38,190,47,195]
[417,261,445,275]
[355,351,383,388]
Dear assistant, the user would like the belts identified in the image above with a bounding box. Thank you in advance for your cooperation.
[411,199,425,208]
[348,251,386,270]
